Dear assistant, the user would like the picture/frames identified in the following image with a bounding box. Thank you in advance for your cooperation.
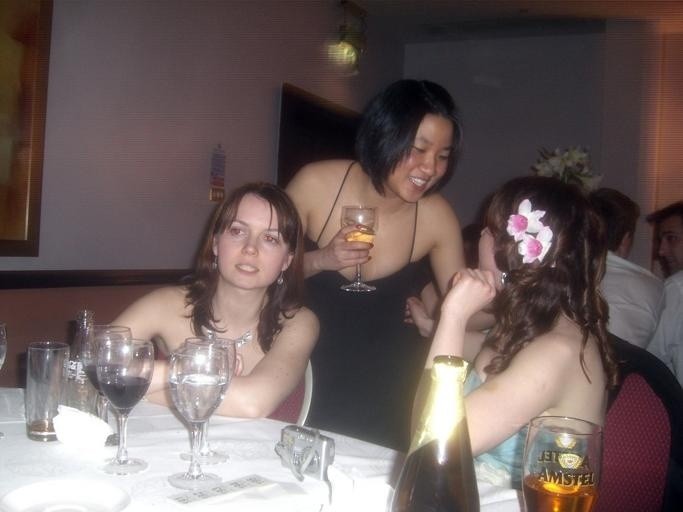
[1,0,53,256]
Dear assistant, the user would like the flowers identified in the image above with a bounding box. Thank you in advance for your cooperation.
[529,142,604,191]
[503,201,554,266]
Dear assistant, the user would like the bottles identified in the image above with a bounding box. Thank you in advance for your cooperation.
[392,355,480,512]
[68,310,100,419]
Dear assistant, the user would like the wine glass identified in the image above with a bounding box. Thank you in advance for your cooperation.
[80,324,236,489]
[0,326,8,437]
[340,208,376,291]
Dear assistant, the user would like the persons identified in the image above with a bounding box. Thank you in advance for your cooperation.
[404,175,625,492]
[97,182,322,424]
[581,186,667,351]
[276,76,497,434]
[647,199,683,394]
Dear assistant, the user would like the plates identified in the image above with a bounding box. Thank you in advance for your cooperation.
[2,480,131,510]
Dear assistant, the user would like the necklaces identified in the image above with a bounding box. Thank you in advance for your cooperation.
[208,331,258,347]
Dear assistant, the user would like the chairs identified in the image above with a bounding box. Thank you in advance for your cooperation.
[590,345,683,511]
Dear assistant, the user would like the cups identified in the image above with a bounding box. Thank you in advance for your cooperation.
[521,416,602,512]
[25,341,70,441]
